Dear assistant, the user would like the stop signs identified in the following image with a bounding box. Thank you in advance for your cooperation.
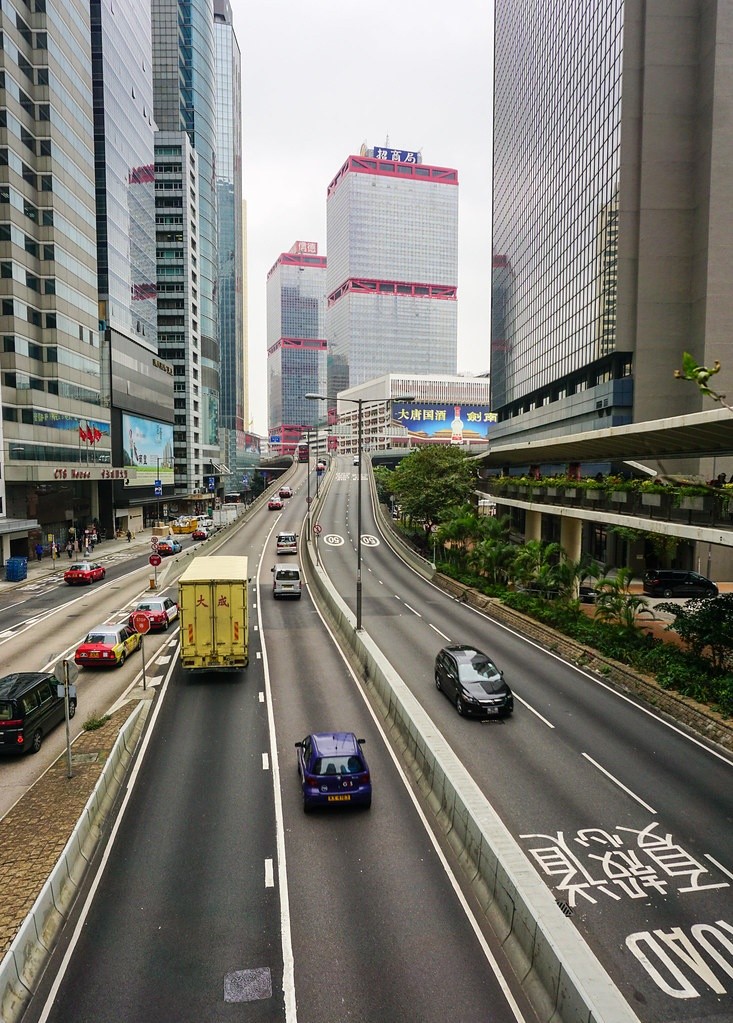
[133,612,150,634]
[148,554,162,566]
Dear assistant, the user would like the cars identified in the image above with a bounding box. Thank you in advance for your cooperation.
[192,520,217,540]
[129,597,179,631]
[268,486,293,510]
[156,539,183,555]
[294,732,373,814]
[315,457,326,471]
[63,562,107,585]
[514,586,603,603]
[75,623,143,669]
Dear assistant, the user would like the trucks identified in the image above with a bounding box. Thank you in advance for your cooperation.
[177,555,251,675]
[212,503,245,531]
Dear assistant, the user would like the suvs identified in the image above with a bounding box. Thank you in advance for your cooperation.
[434,643,514,718]
[0,672,78,753]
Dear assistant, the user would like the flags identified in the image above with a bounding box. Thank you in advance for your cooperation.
[79,427,102,445]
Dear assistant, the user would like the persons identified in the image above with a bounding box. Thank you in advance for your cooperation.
[713,472,733,488]
[50,536,82,558]
[35,543,43,561]
[97,532,101,543]
[126,530,131,542]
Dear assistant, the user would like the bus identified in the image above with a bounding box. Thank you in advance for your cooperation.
[293,443,309,463]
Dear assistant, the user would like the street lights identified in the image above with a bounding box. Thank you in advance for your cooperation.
[305,393,415,629]
[284,427,332,541]
[301,424,333,498]
[156,457,175,527]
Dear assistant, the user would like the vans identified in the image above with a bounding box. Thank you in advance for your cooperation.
[271,563,302,600]
[642,568,719,597]
[275,531,299,555]
[353,453,359,466]
